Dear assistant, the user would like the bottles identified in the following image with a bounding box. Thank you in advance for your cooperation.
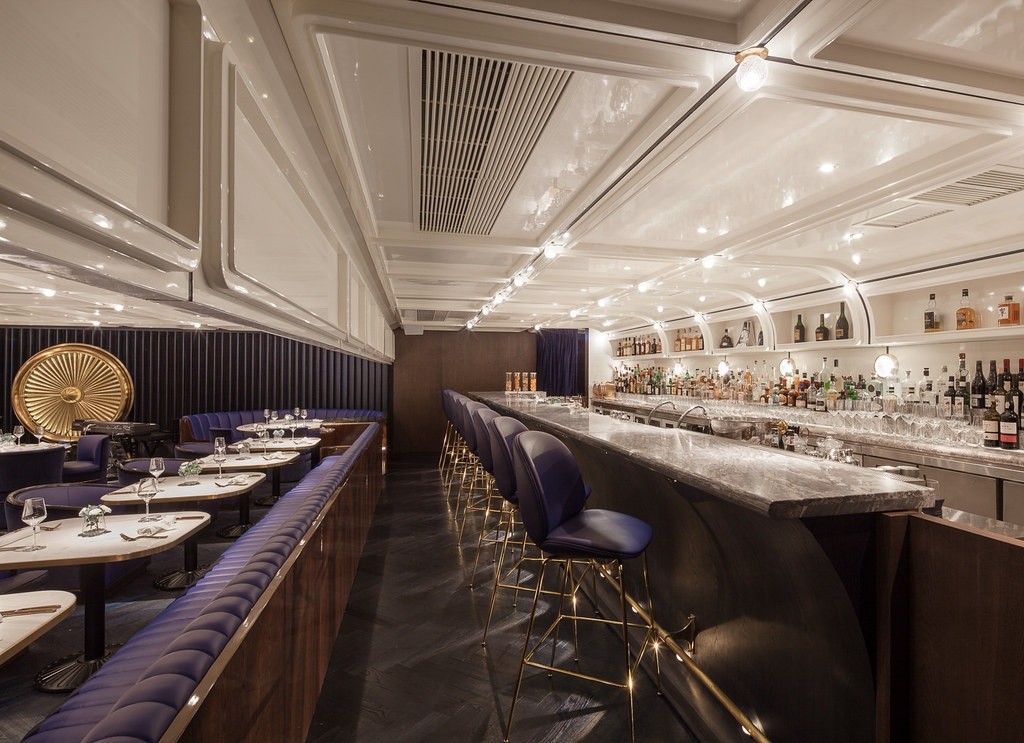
[590,287,1024,467]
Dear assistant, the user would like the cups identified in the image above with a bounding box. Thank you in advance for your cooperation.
[872,464,940,501]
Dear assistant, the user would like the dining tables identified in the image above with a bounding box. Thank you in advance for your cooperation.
[0,418,328,693]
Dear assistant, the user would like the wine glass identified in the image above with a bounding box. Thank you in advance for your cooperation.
[0,409,309,552]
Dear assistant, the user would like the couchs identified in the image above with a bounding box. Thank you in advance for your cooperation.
[17,421,385,742]
[179,408,387,453]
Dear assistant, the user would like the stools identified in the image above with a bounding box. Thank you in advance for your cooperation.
[437,387,662,743]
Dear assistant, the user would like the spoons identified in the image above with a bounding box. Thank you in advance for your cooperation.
[1,605,61,618]
